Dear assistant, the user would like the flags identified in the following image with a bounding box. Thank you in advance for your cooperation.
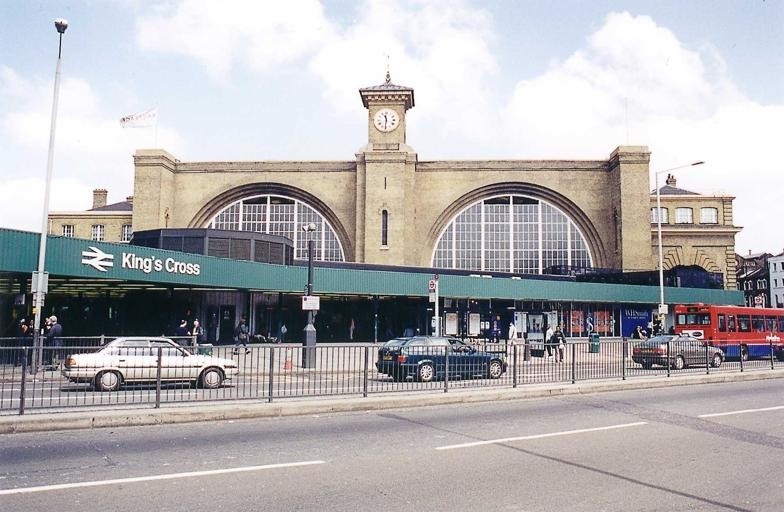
[120,105,153,129]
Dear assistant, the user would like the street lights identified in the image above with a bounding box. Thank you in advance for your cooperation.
[654,160,704,330]
[301,223,317,322]
[28,19,68,371]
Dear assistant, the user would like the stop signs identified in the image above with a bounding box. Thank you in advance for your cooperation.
[428,280,435,289]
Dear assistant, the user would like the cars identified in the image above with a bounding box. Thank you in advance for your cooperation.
[632,333,725,369]
[376,336,506,380]
[63,336,241,389]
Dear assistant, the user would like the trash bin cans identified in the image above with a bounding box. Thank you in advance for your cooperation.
[589,333,600,352]
[199,343,213,355]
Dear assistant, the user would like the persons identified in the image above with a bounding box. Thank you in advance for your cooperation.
[174,319,191,346]
[545,324,553,356]
[192,318,204,344]
[234,319,247,347]
[551,326,566,363]
[508,322,517,340]
[670,326,675,334]
[16,315,62,371]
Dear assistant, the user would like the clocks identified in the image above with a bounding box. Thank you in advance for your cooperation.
[374,109,401,134]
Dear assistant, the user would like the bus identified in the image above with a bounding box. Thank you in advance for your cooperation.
[674,303,784,361]
